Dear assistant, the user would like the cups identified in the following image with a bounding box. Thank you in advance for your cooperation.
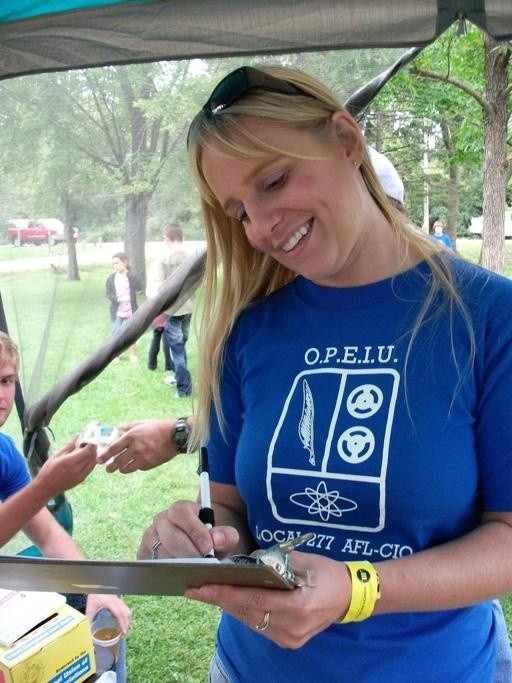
[88,616,123,679]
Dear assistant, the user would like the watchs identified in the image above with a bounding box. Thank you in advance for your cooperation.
[171,417,191,454]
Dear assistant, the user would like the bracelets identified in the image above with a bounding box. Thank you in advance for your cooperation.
[336,560,380,626]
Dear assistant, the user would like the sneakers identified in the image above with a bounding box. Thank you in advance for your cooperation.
[165,376,179,399]
[114,354,139,363]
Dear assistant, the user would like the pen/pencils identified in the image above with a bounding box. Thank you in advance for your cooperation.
[199,446,215,558]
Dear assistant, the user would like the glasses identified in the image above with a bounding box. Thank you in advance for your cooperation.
[186,67,315,149]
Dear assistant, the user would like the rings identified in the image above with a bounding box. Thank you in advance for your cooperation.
[256,613,270,631]
[151,540,162,559]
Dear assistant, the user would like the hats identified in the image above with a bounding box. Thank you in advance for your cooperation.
[367,145,409,202]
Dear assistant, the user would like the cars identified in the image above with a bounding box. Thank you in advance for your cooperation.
[7,218,79,245]
[470,211,512,239]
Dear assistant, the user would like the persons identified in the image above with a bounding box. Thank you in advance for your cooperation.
[105,222,196,399]
[431,219,453,250]
[136,64,512,683]
[94,145,407,474]
[0,332,133,638]
